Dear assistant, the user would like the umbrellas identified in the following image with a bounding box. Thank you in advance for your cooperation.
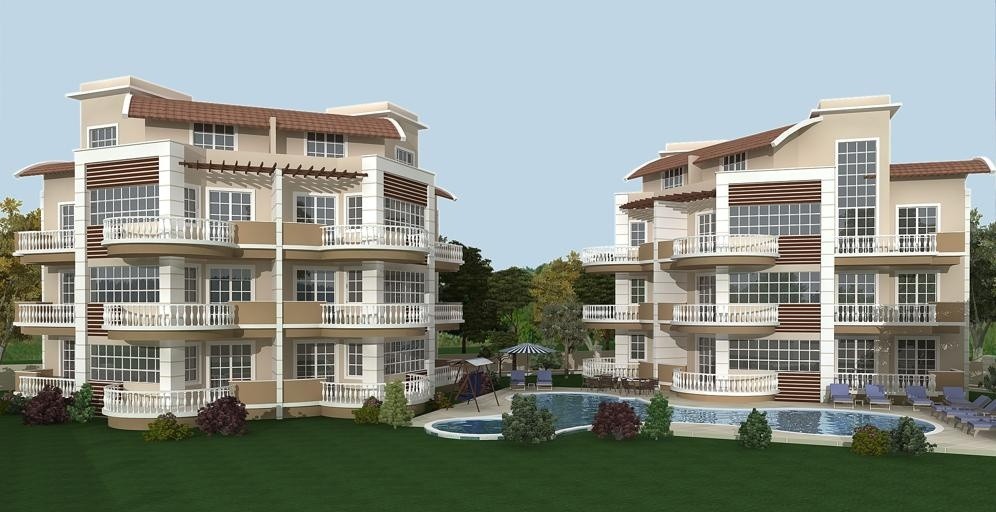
[498,341,561,378]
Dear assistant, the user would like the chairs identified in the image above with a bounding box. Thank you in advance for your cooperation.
[582,373,659,396]
[830,383,996,439]
[509,369,553,392]
